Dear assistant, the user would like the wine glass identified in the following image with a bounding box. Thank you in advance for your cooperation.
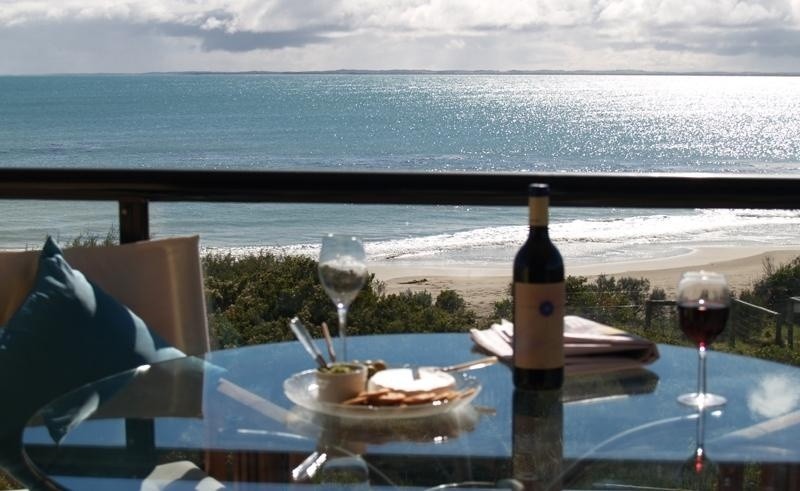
[319,233,368,363]
[676,270,730,407]
[681,410,720,490]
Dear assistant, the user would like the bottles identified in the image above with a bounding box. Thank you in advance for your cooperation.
[512,388,564,491]
[513,183,566,387]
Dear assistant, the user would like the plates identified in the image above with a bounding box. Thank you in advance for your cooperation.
[284,366,477,424]
